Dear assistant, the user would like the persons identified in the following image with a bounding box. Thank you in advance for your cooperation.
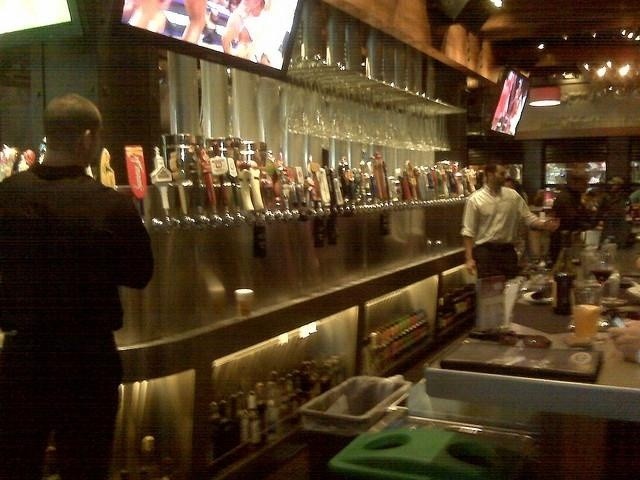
[121,0,208,45]
[0,92,156,480]
[220,0,278,66]
[608,320,640,363]
[459,158,640,329]
[497,76,526,134]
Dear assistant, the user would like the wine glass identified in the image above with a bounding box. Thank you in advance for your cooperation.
[591,266,614,303]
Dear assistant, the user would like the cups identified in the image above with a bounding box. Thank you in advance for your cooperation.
[234,288,256,318]
[604,243,618,265]
[572,304,602,342]
[575,287,599,307]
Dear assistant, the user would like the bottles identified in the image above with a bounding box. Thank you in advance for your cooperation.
[437,285,475,330]
[43,434,176,480]
[361,308,431,374]
[544,187,553,209]
[209,354,342,472]
[608,270,620,299]
[570,259,585,293]
[553,272,576,316]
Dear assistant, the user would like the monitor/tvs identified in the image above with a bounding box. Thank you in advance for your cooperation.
[487,68,531,137]
[110,0,303,78]
[0,0,88,48]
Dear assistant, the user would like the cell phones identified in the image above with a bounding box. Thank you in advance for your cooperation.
[609,316,626,327]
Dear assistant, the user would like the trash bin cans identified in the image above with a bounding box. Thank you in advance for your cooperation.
[328,430,505,480]
[297,375,413,480]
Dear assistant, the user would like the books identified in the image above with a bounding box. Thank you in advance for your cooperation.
[439,342,603,384]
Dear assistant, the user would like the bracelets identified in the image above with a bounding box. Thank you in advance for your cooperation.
[464,255,474,260]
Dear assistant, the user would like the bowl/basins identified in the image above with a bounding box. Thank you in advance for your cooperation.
[625,288,640,304]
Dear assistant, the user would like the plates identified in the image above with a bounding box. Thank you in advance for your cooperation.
[522,290,555,305]
